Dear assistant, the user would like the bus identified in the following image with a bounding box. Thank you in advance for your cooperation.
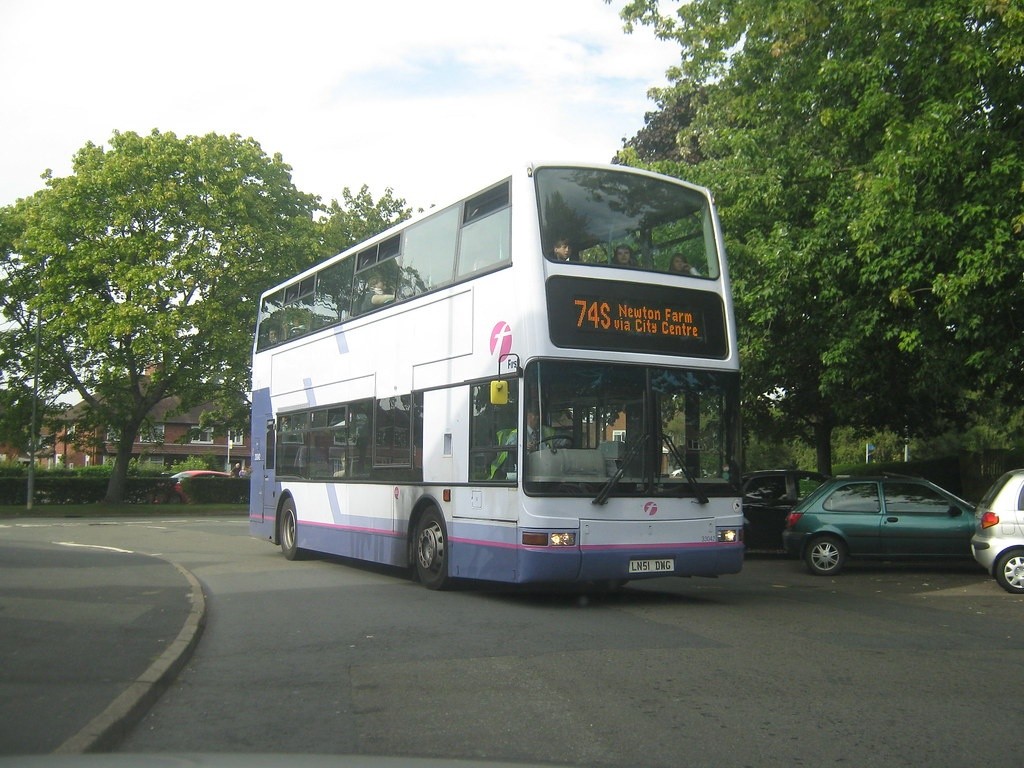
[247,160,749,591]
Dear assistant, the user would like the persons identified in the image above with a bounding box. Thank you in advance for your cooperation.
[231,460,251,479]
[505,412,546,472]
[548,236,575,263]
[669,253,702,276]
[613,244,638,266]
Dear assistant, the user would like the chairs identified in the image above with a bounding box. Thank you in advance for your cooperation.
[295,445,331,477]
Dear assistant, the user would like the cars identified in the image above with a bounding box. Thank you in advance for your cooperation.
[781,477,977,576]
[145,470,232,503]
[971,469,1024,594]
[726,469,878,559]
[670,469,683,478]
